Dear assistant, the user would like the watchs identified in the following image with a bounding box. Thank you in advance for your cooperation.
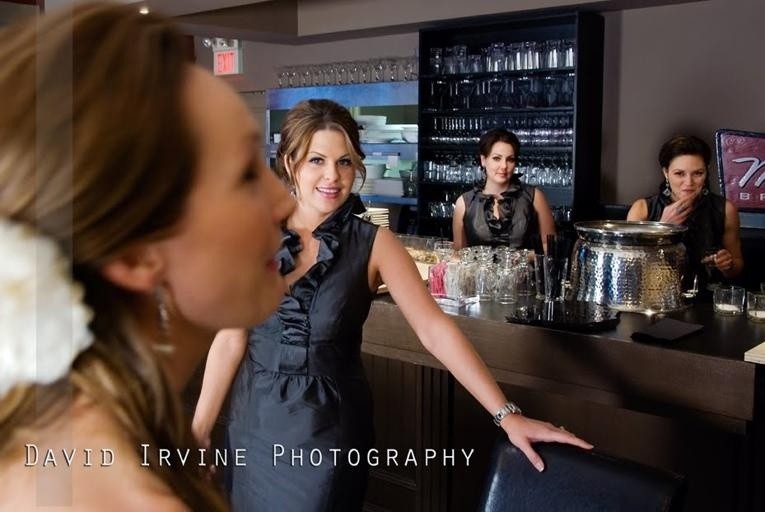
[493,402,522,427]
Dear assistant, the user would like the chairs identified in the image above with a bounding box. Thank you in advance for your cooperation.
[483,442,689,512]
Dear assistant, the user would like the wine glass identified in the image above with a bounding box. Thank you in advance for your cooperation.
[426,115,573,148]
[442,246,520,299]
[494,248,519,304]
[273,58,420,83]
[424,155,573,188]
[474,246,497,302]
[434,241,455,268]
[515,250,535,296]
[425,73,574,108]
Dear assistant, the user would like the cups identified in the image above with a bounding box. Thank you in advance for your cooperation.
[747,288,765,322]
[543,255,568,303]
[429,37,577,75]
[549,204,573,224]
[533,254,545,301]
[426,200,457,219]
[713,285,745,314]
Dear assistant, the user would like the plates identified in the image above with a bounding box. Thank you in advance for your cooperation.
[356,115,418,145]
[359,203,390,231]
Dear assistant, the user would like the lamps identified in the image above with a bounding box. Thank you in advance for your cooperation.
[200,37,244,79]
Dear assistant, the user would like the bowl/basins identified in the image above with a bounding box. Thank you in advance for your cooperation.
[363,163,386,180]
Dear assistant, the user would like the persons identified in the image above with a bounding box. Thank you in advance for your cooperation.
[627,134,744,288]
[1,2,295,512]
[190,98,594,511]
[453,128,558,257]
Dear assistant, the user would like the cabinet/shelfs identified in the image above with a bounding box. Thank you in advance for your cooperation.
[413,8,607,245]
[265,79,420,237]
